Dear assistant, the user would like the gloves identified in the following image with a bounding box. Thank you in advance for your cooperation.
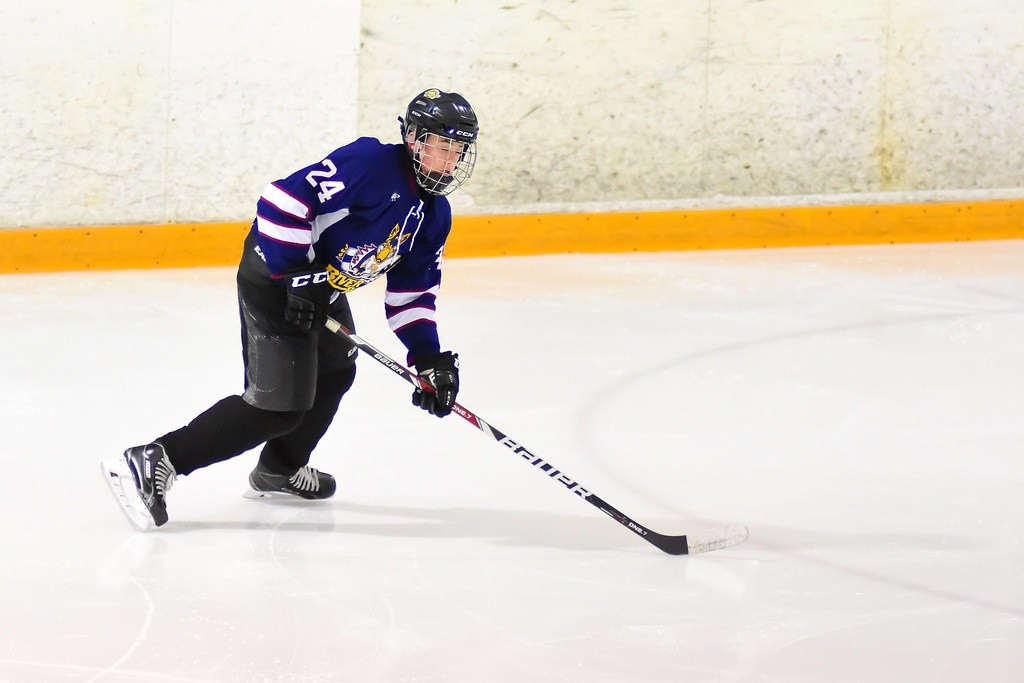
[412,352,460,418]
[283,269,330,333]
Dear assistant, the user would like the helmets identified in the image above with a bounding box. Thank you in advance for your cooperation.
[398,88,479,196]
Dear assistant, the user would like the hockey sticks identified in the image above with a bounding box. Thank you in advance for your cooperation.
[325,313,749,556]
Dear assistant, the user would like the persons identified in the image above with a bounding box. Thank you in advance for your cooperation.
[100,87,481,532]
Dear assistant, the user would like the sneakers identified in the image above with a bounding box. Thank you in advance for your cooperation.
[242,461,338,504]
[101,443,179,532]
